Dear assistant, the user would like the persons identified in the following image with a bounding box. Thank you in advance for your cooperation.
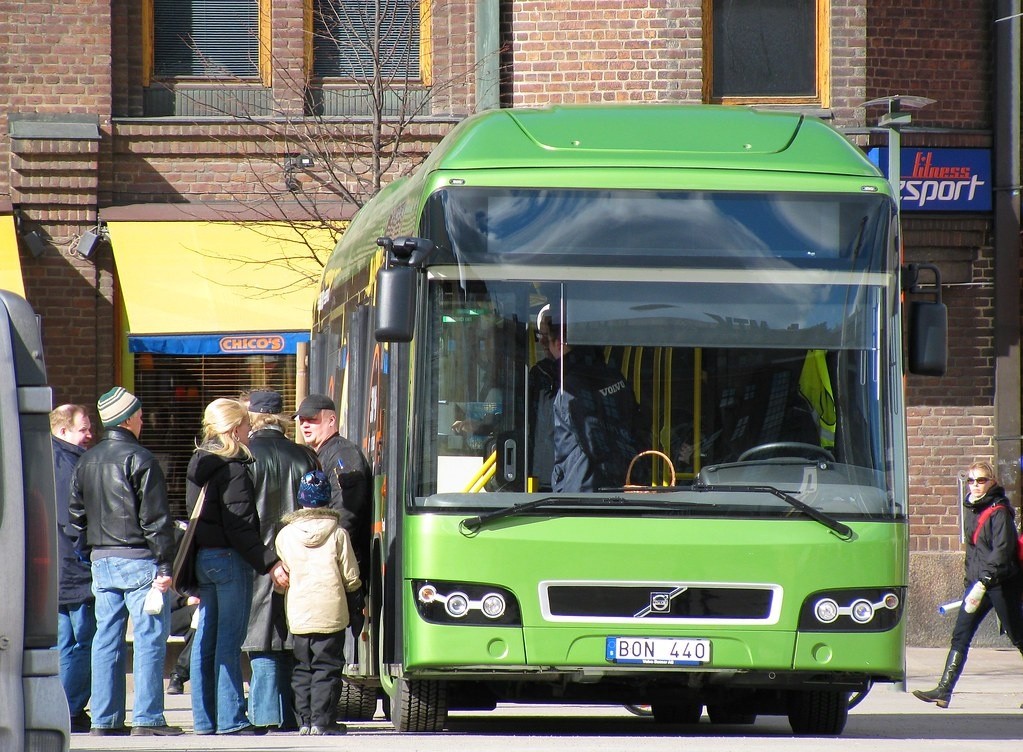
[238,388,322,736]
[913,462,1023,709]
[454,304,644,493]
[681,348,819,468]
[166,587,200,693]
[70,387,184,737]
[273,470,363,736]
[185,398,290,734]
[290,393,372,635]
[49,403,93,733]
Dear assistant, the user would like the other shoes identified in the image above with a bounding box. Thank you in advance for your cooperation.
[227,724,267,735]
[310,721,347,735]
[300,725,310,735]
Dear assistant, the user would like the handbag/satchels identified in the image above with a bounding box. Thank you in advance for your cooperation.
[171,519,199,598]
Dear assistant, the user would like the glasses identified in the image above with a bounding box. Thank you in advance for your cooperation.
[535,332,548,340]
[967,477,992,484]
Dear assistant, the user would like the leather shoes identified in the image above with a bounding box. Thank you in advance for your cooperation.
[130,723,185,736]
[88,724,133,736]
[166,673,183,693]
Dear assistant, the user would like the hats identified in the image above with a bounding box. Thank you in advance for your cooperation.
[291,394,335,419]
[248,392,281,413]
[97,386,142,428]
[297,470,331,507]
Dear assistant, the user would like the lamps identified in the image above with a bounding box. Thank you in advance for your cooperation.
[285,155,314,192]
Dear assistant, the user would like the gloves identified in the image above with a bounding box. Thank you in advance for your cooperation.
[965,581,987,614]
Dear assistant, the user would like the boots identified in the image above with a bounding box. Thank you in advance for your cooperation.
[913,649,965,708]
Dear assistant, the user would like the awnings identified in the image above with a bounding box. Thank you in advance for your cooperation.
[107,221,351,355]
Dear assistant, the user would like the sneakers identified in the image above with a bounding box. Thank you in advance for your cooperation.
[71,707,91,732]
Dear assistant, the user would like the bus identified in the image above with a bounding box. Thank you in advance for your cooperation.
[306,102,949,736]
